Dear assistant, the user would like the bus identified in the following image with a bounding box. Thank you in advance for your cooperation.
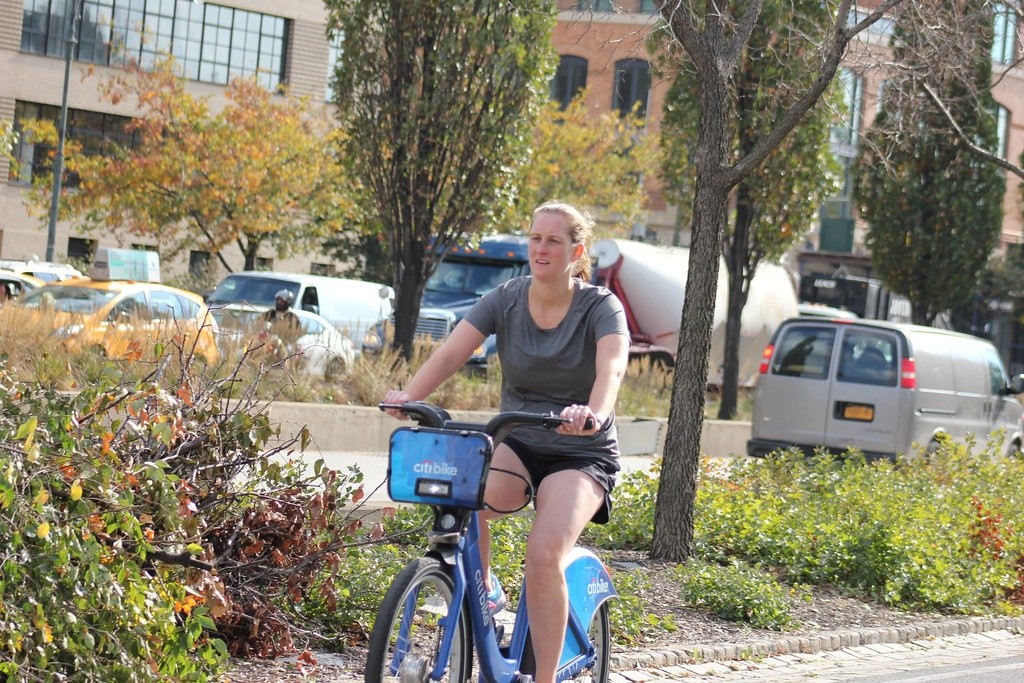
[415,231,532,303]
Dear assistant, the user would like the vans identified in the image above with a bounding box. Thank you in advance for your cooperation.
[743,314,1024,470]
[201,271,396,348]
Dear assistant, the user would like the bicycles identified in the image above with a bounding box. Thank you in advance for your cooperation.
[364,399,622,682]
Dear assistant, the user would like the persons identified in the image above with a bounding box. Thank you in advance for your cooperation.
[258,288,303,363]
[383,201,632,683]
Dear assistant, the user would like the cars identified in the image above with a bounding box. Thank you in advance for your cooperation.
[0,267,56,315]
[0,246,221,381]
[358,294,496,365]
[0,259,84,285]
[208,302,360,383]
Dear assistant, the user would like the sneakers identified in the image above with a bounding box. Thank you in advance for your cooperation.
[484,573,507,616]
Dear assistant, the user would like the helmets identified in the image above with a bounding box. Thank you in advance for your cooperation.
[274,288,294,306]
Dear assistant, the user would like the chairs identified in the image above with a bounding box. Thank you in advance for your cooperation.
[856,346,889,369]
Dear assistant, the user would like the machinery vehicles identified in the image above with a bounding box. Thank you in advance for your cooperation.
[590,236,910,392]
